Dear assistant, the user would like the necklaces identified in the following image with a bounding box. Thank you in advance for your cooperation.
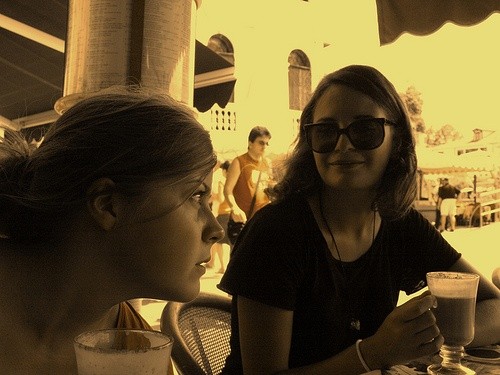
[318,186,376,331]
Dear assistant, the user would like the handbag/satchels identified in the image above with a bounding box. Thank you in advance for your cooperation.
[227,217,246,247]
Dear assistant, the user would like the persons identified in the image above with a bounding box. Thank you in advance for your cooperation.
[216,65,500,375]
[203,160,231,273]
[0,86,225,375]
[223,126,274,253]
[431,177,461,233]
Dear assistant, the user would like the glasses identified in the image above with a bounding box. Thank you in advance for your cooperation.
[303,117,394,154]
[254,140,270,146]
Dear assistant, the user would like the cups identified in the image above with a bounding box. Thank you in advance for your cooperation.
[73,329,174,375]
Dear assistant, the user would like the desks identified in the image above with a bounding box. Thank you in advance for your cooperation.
[413,202,437,228]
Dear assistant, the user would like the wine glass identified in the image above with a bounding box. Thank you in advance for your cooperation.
[425,271,480,375]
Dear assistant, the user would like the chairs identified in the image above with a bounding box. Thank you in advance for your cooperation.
[160,292,239,375]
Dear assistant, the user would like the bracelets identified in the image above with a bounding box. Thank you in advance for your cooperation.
[356,339,371,372]
